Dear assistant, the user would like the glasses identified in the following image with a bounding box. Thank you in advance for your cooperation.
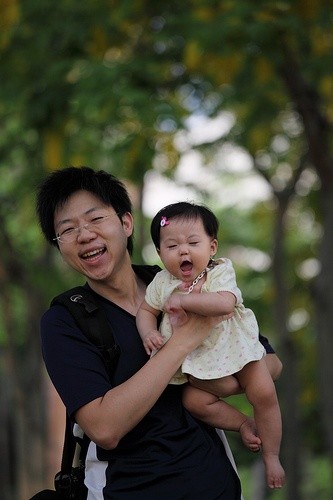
[53,213,120,244]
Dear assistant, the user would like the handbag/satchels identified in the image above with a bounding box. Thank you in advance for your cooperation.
[29,472,93,500]
